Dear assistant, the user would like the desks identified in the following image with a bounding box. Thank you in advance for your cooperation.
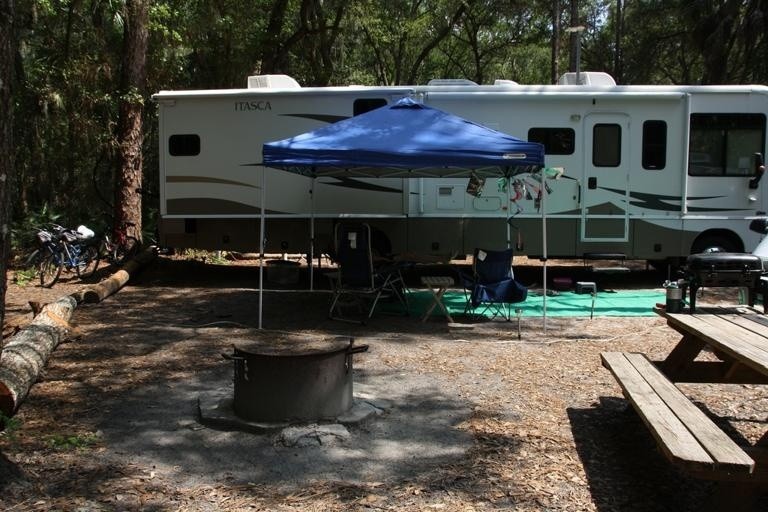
[653,304,768,511]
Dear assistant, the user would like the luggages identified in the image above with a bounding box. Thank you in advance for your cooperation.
[683,253,763,285]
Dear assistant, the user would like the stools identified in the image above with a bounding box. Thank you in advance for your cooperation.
[324,271,364,316]
[419,276,455,324]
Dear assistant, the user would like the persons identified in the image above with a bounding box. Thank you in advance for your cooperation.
[352,238,401,316]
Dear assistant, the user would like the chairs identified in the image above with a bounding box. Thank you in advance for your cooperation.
[325,223,409,326]
[462,248,528,322]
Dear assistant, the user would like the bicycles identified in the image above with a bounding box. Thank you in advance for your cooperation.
[31,222,139,288]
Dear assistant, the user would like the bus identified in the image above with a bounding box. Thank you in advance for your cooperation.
[151,71,768,288]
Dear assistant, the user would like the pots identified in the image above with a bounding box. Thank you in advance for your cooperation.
[224,337,369,361]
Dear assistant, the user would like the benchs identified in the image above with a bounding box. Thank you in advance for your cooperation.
[601,351,757,511]
